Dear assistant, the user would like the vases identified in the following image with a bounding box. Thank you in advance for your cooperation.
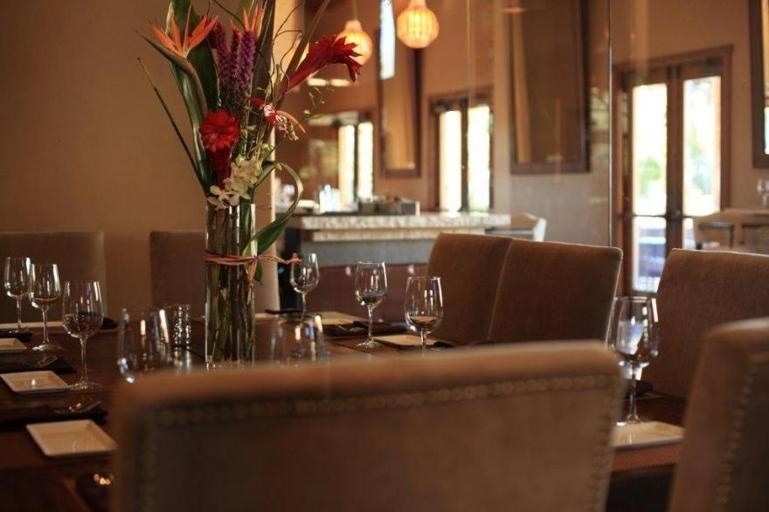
[203,202,257,367]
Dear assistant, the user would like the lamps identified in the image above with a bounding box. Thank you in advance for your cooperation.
[333,0,440,67]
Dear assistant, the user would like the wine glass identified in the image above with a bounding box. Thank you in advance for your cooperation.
[757,179,769,210]
[605,296,660,435]
[291,252,320,323]
[4,256,32,333]
[404,276,444,353]
[27,262,64,356]
[61,278,105,394]
[354,260,389,352]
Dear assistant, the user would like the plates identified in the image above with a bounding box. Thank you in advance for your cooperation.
[0,371,69,395]
[0,338,27,354]
[25,419,121,457]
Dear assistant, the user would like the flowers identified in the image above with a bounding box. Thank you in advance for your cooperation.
[134,3,363,367]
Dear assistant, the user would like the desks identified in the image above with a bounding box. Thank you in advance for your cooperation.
[2,311,684,508]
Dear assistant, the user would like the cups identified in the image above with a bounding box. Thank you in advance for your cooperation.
[117,306,174,384]
[271,311,326,368]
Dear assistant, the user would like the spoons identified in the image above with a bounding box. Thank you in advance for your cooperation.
[0,399,104,416]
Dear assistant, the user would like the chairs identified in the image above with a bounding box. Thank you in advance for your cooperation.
[669,313,769,512]
[0,230,107,318]
[729,227,768,256]
[486,242,627,349]
[417,231,516,343]
[637,246,766,430]
[96,334,630,511]
[150,226,212,325]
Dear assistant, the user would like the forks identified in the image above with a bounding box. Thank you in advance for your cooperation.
[0,353,59,369]
